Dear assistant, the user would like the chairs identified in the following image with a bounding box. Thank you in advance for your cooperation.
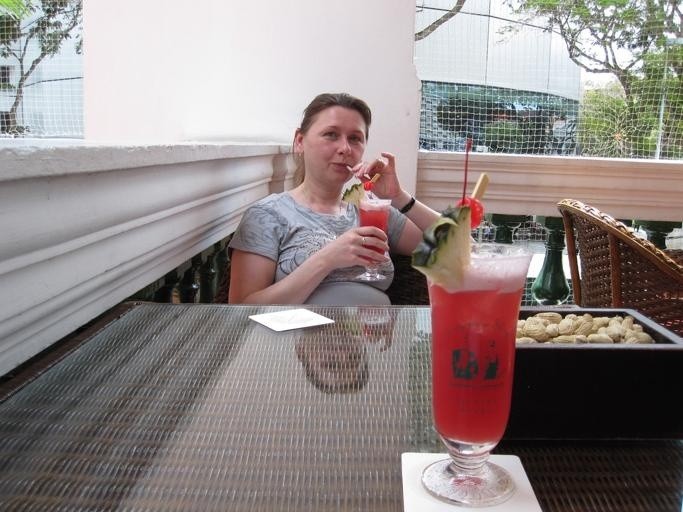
[556,199,681,342]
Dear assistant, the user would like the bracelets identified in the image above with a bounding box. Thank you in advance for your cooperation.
[396,194,417,214]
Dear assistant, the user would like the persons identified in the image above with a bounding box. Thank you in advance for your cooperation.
[291,309,405,394]
[223,91,443,308]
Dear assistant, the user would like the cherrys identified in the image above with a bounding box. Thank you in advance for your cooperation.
[457,196,483,229]
[364,181,373,191]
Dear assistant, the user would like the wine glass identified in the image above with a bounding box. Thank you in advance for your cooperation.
[354,197,393,284]
[415,242,534,508]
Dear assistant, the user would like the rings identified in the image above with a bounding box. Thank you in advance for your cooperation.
[363,236,368,245]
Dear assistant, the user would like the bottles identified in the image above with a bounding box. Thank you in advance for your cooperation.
[153,232,235,303]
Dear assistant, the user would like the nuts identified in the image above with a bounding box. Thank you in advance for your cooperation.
[516,311,655,343]
